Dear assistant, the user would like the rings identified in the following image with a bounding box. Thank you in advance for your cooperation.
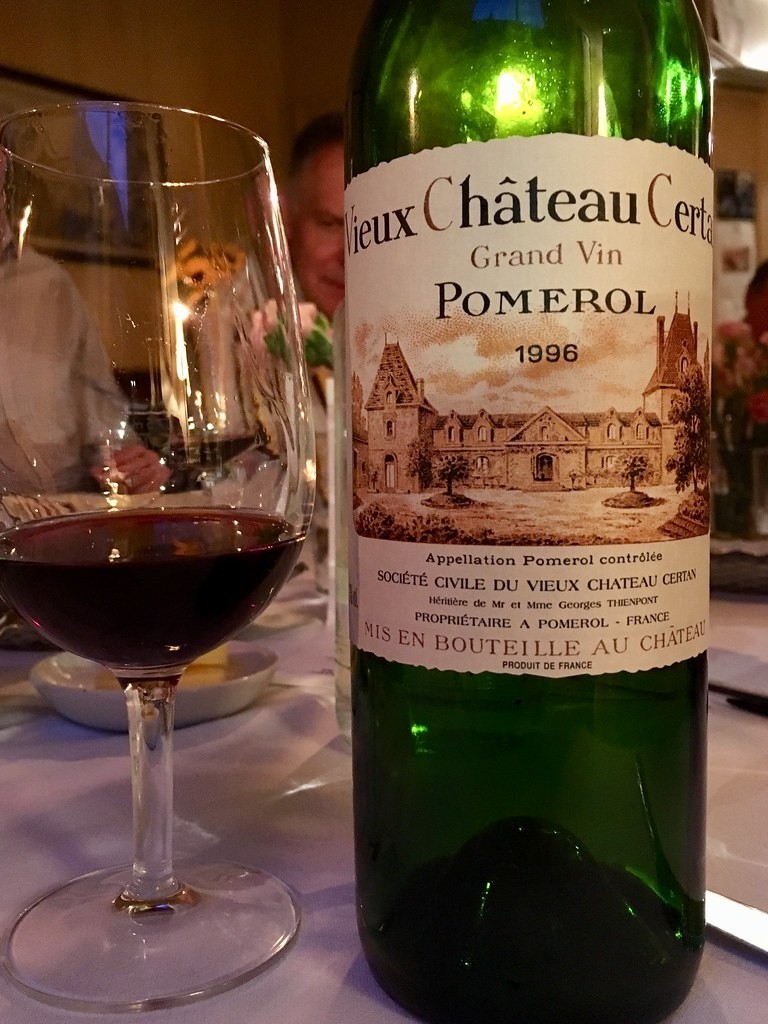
[124,477,132,487]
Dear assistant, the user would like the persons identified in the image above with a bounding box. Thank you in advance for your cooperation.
[191,110,344,470]
[0,216,170,496]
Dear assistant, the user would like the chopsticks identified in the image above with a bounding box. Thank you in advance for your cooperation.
[708,682,768,717]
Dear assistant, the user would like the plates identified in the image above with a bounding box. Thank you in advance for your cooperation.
[29,638,277,733]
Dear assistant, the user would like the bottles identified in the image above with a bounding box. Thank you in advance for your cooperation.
[341,1,718,1024]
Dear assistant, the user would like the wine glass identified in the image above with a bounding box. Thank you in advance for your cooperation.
[1,101,316,1012]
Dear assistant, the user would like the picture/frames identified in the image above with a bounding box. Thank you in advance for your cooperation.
[0,63,168,266]
